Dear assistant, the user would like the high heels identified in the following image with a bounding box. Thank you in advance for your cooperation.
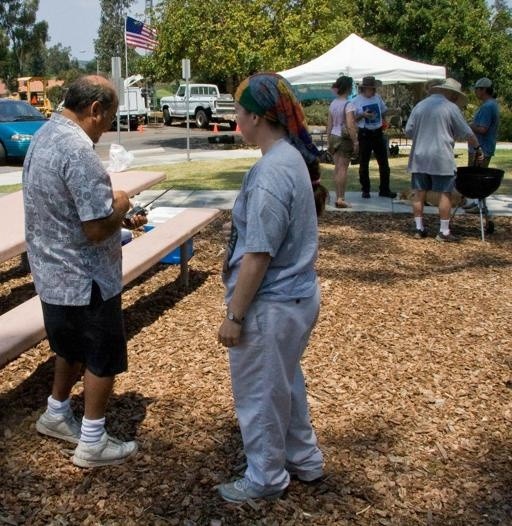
[336,197,354,208]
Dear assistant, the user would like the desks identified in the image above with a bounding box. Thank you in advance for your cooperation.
[308,131,329,151]
[1,169,168,262]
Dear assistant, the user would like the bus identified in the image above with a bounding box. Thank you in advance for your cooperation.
[293,81,358,106]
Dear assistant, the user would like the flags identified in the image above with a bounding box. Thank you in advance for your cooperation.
[126,16,159,50]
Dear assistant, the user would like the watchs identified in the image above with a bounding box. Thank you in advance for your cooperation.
[227,311,242,324]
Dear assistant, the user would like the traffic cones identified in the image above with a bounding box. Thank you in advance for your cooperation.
[212,125,217,134]
[235,123,240,135]
[139,124,144,132]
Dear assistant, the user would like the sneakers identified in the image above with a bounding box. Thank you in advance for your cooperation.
[72,429,139,469]
[462,202,488,214]
[362,186,396,198]
[36,407,82,443]
[436,231,460,243]
[219,477,285,502]
[413,227,429,239]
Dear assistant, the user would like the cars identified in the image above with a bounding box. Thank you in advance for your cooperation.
[0,99,50,161]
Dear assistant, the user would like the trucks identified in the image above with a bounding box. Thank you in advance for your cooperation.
[55,86,150,131]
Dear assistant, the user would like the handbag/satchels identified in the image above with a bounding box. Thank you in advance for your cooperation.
[341,126,361,159]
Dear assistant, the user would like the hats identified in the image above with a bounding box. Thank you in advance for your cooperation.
[359,77,381,88]
[424,77,468,108]
[473,77,494,89]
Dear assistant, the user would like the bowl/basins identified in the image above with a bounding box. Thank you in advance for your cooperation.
[451,166,506,198]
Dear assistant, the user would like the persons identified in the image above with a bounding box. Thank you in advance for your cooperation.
[23,75,146,467]
[351,76,397,198]
[462,77,500,214]
[405,78,485,241]
[218,71,331,504]
[327,76,359,208]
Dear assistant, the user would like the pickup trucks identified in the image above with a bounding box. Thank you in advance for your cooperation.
[160,83,240,130]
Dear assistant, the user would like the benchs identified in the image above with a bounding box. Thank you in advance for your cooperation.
[0,204,220,362]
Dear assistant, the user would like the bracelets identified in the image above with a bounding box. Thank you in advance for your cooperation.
[474,145,481,150]
